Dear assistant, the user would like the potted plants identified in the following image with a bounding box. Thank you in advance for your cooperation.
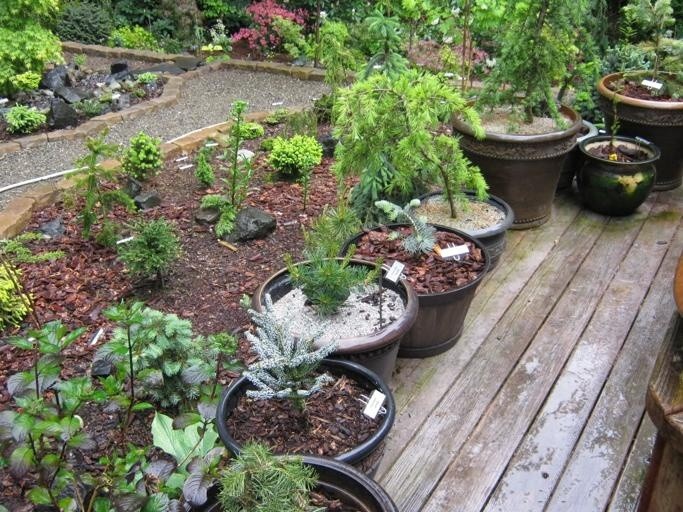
[177,0,682,512]
